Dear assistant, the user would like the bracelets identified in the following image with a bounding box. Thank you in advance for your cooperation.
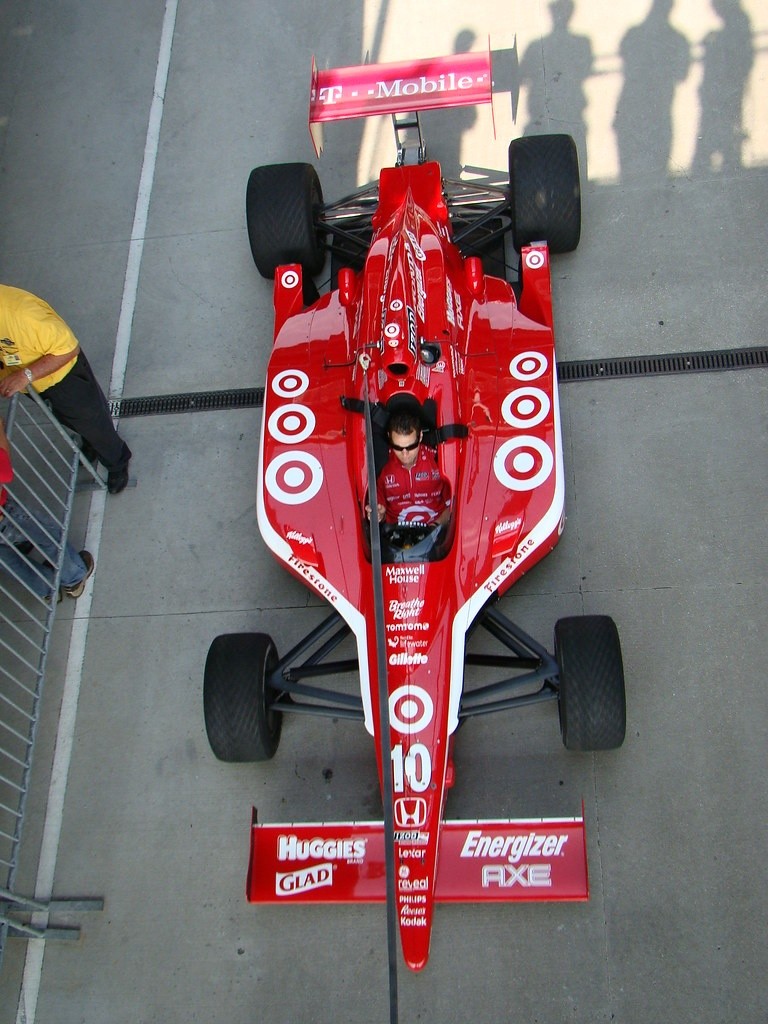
[24,367,35,382]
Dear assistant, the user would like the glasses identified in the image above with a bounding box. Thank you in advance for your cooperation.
[389,430,420,451]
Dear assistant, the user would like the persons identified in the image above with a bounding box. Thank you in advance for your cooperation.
[0,284,132,494]
[365,410,451,563]
[0,422,94,604]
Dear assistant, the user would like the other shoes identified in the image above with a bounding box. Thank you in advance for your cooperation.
[108,442,132,495]
[78,441,96,465]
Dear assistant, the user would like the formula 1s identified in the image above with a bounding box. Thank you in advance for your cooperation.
[201,32,629,973]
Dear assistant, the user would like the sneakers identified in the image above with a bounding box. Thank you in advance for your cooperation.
[40,560,63,604]
[64,550,94,598]
